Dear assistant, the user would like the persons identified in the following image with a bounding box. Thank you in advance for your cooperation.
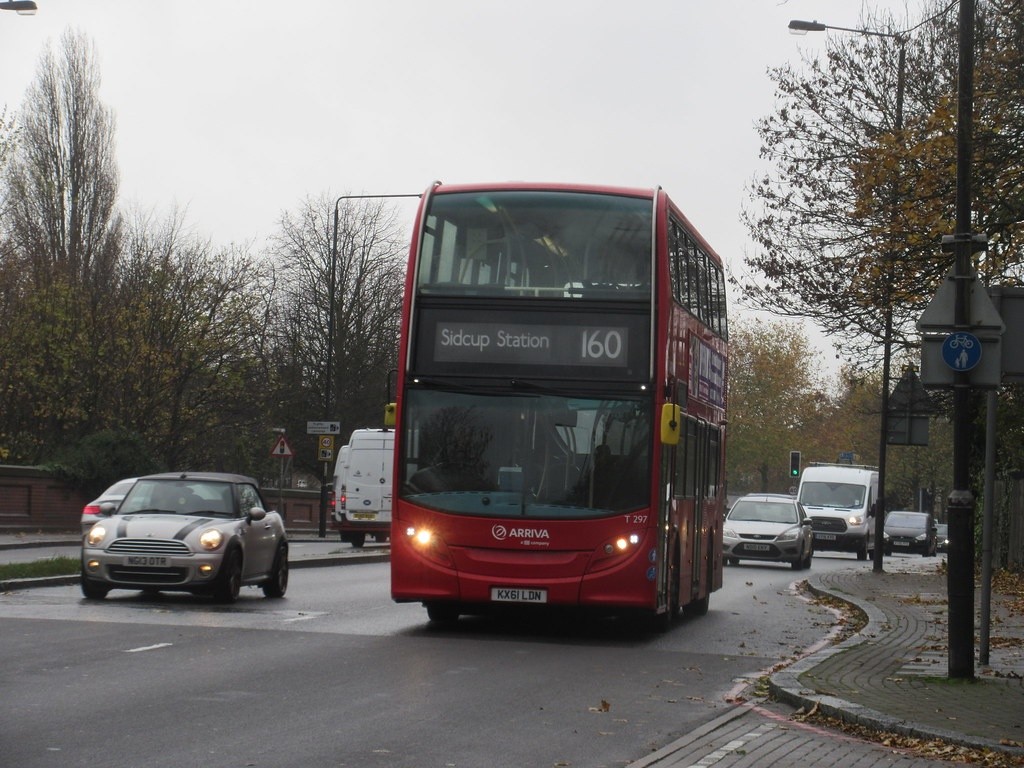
[580,445,612,491]
[432,422,472,465]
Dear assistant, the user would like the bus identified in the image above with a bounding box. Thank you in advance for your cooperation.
[390,180,728,629]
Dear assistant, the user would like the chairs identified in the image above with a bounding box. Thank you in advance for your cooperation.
[539,462,581,492]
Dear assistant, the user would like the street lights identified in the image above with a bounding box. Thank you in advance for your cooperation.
[788,20,907,571]
[318,193,423,539]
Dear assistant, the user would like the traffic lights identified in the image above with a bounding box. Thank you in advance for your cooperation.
[789,451,800,478]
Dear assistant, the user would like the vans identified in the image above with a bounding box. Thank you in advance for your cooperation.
[332,428,395,547]
[796,467,879,560]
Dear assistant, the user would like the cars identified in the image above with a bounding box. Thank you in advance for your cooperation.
[80,472,289,601]
[723,493,814,570]
[883,511,948,557]
